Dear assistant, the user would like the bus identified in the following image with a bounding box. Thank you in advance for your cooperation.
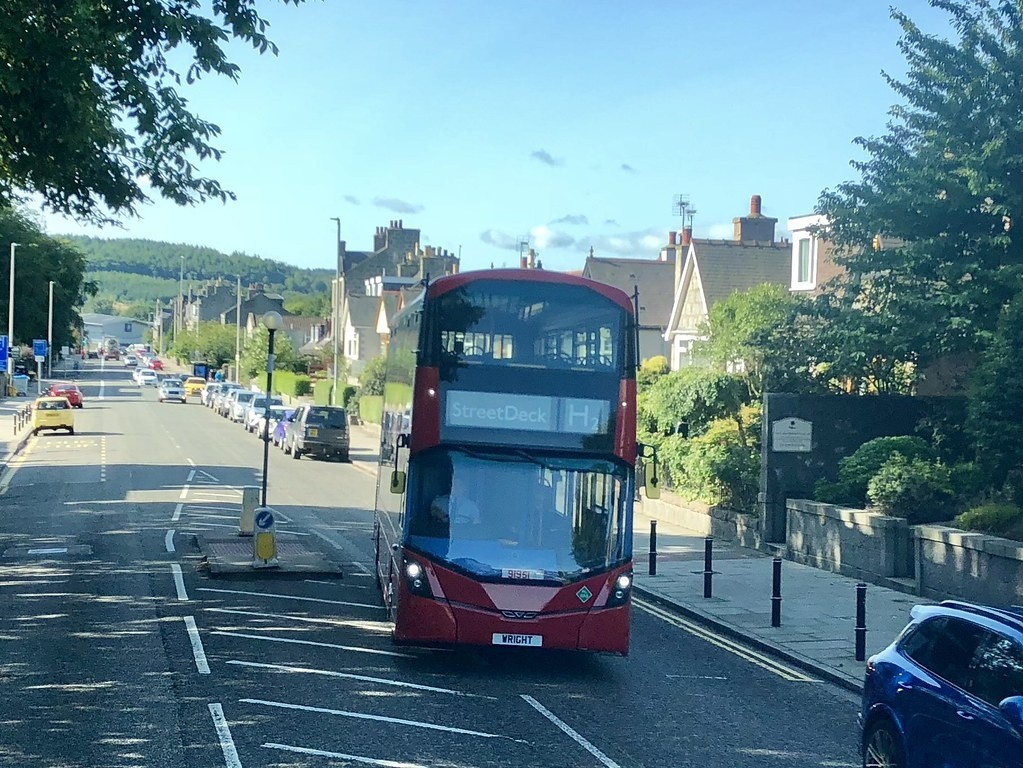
[372,267,661,655]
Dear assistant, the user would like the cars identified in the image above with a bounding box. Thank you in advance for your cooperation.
[200,382,259,424]
[178,373,194,383]
[47,383,83,409]
[30,397,75,436]
[272,410,296,450]
[257,405,295,441]
[184,376,206,397]
[158,379,186,404]
[119,343,163,381]
[137,370,158,389]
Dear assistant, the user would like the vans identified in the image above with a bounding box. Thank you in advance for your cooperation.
[283,404,350,462]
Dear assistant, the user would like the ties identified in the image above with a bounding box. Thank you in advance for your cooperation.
[450,498,457,523]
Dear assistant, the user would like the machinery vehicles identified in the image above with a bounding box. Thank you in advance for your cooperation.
[103,338,120,360]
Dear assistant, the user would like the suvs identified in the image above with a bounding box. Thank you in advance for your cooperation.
[857,598,1023,768]
[244,393,285,434]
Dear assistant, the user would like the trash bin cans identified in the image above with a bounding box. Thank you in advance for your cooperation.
[13,375,30,396]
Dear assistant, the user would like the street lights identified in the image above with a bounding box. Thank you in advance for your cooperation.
[329,216,341,405]
[47,280,57,378]
[262,309,284,507]
[8,242,38,385]
[232,274,240,381]
[179,255,185,331]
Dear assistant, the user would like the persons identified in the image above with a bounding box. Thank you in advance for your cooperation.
[431,476,480,525]
